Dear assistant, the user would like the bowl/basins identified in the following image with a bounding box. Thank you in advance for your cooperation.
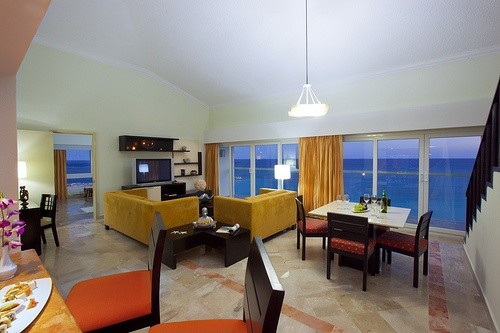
[0,299,24,314]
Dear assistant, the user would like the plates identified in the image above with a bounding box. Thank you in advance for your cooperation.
[0,278,52,333]
[352,207,370,213]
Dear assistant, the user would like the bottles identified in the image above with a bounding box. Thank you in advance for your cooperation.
[196,208,213,224]
[380,190,387,213]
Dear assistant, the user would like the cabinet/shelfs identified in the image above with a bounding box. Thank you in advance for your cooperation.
[119,135,203,178]
[120,182,186,201]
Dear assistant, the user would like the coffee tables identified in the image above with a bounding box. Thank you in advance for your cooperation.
[198,202,214,217]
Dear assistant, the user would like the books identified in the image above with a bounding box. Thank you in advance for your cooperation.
[216,224,240,234]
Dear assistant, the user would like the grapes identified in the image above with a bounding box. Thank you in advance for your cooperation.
[354,203,366,211]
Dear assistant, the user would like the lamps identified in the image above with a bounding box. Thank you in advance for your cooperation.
[287,0,328,117]
[17,160,30,210]
[273,165,291,191]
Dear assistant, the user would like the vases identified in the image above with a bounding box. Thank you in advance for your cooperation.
[0,245,17,282]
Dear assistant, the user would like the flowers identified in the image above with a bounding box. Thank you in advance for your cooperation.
[0,193,27,250]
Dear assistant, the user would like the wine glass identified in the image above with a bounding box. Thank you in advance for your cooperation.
[364,193,370,205]
[337,195,342,209]
[343,195,349,209]
[371,194,377,204]
[368,201,384,219]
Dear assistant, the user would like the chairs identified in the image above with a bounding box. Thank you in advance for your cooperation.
[64,211,165,333]
[37,194,61,246]
[148,235,284,333]
[296,195,434,288]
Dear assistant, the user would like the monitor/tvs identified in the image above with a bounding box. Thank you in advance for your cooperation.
[131,158,174,186]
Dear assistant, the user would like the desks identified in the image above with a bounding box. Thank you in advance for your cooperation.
[84,187,93,197]
[18,203,43,256]
[310,200,411,272]
[163,220,252,269]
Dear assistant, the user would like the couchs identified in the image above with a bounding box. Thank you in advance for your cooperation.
[104,189,200,249]
[212,189,296,242]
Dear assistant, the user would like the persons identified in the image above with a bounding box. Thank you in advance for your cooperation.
[198,208,213,222]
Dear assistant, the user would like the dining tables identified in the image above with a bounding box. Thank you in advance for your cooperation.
[0,250,84,333]
